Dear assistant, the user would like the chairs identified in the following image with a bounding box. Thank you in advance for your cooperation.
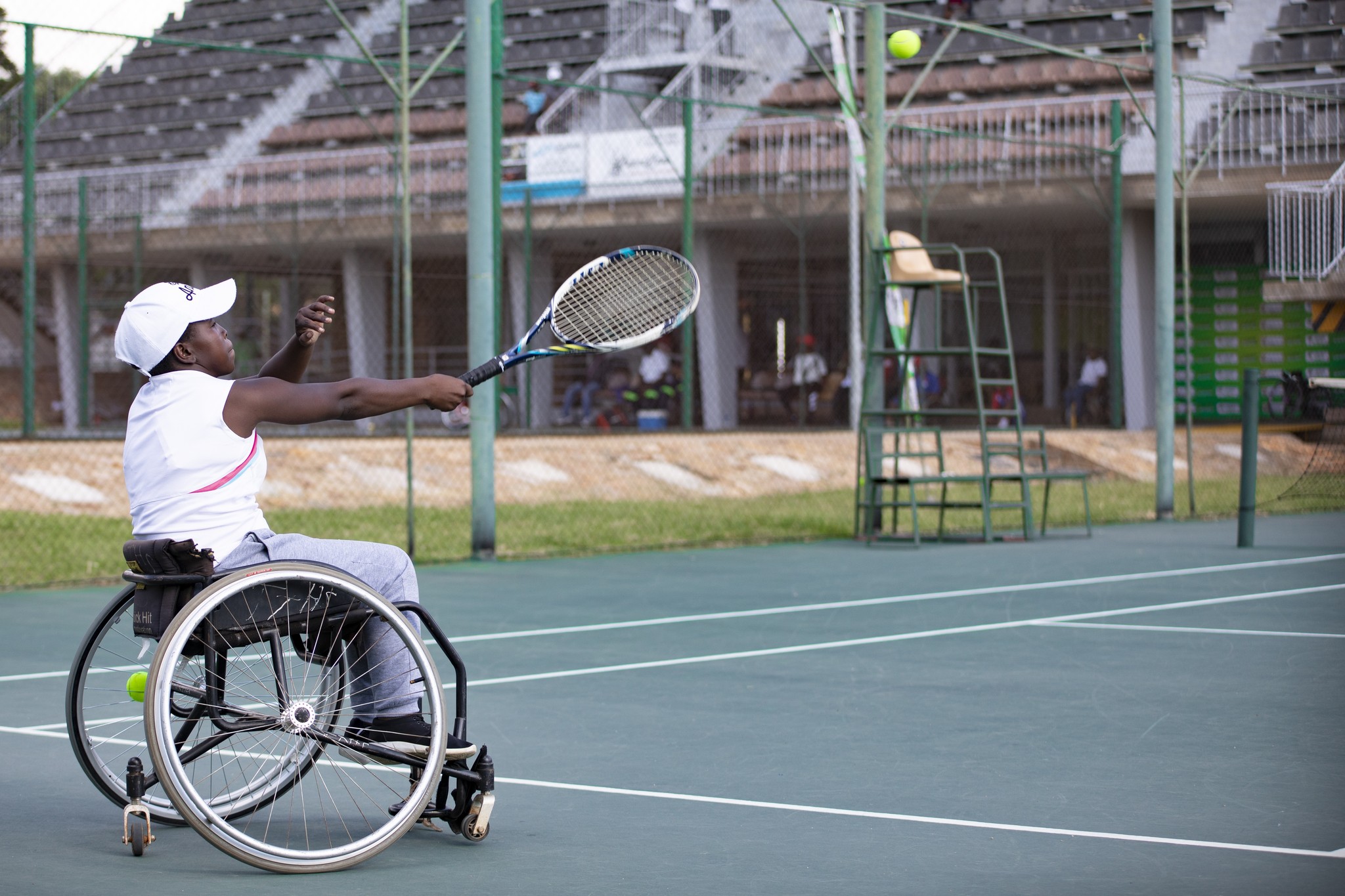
[887,228,973,293]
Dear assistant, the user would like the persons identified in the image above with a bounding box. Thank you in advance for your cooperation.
[877,347,1025,429]
[777,336,825,425]
[516,80,547,135]
[831,349,850,426]
[1063,347,1109,425]
[552,340,669,429]
[736,324,750,387]
[115,278,480,759]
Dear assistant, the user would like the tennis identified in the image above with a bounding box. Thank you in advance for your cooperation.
[127,671,149,703]
[887,30,921,58]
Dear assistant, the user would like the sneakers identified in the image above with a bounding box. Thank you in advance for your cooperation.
[368,711,477,761]
[338,717,404,766]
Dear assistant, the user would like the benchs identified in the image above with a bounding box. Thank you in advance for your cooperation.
[0,0,608,230]
[711,1,1345,181]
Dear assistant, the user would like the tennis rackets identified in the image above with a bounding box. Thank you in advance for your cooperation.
[425,244,701,411]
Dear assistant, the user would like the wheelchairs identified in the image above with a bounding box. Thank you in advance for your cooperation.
[64,537,495,875]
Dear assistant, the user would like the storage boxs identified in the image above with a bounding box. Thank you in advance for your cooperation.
[638,410,667,431]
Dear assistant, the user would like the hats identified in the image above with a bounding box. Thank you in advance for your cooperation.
[114,277,237,378]
[796,335,815,346]
